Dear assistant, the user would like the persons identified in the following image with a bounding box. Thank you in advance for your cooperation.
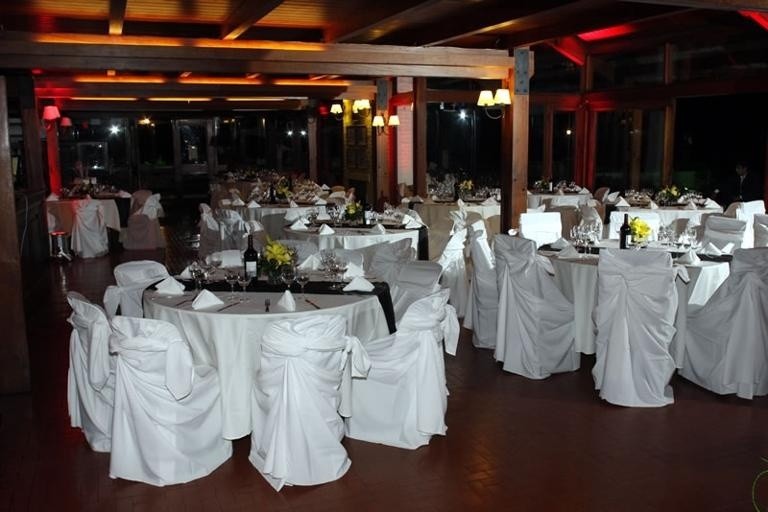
[710,156,756,203]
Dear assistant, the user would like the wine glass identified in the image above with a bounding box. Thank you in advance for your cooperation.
[688,190,702,206]
[384,204,404,224]
[624,185,654,199]
[661,192,670,208]
[569,224,600,254]
[188,247,349,304]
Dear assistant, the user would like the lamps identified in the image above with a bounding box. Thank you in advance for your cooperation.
[476,88,511,120]
[39,106,60,130]
[372,115,400,136]
[59,117,72,135]
[330,104,344,120]
[352,99,371,119]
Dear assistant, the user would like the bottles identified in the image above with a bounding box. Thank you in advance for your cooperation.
[453,179,460,201]
[363,194,373,226]
[244,234,258,292]
[620,214,632,250]
[270,183,278,204]
[548,174,555,194]
